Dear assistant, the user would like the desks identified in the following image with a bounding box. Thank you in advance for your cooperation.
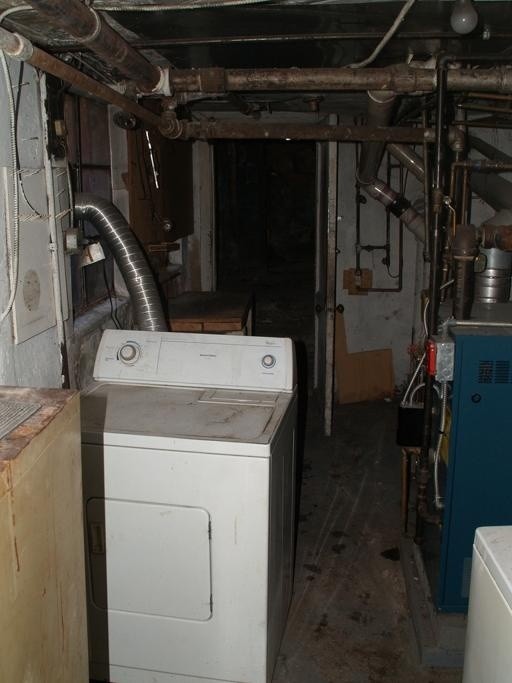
[162,290,256,336]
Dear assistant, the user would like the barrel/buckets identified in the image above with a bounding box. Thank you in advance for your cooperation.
[476,248,511,303]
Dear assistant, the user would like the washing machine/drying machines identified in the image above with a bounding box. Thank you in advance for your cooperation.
[78,331,300,682]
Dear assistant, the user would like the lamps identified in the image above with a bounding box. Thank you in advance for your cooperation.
[450,0,479,35]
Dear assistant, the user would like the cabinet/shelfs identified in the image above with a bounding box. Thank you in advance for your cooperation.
[0,383,91,683]
